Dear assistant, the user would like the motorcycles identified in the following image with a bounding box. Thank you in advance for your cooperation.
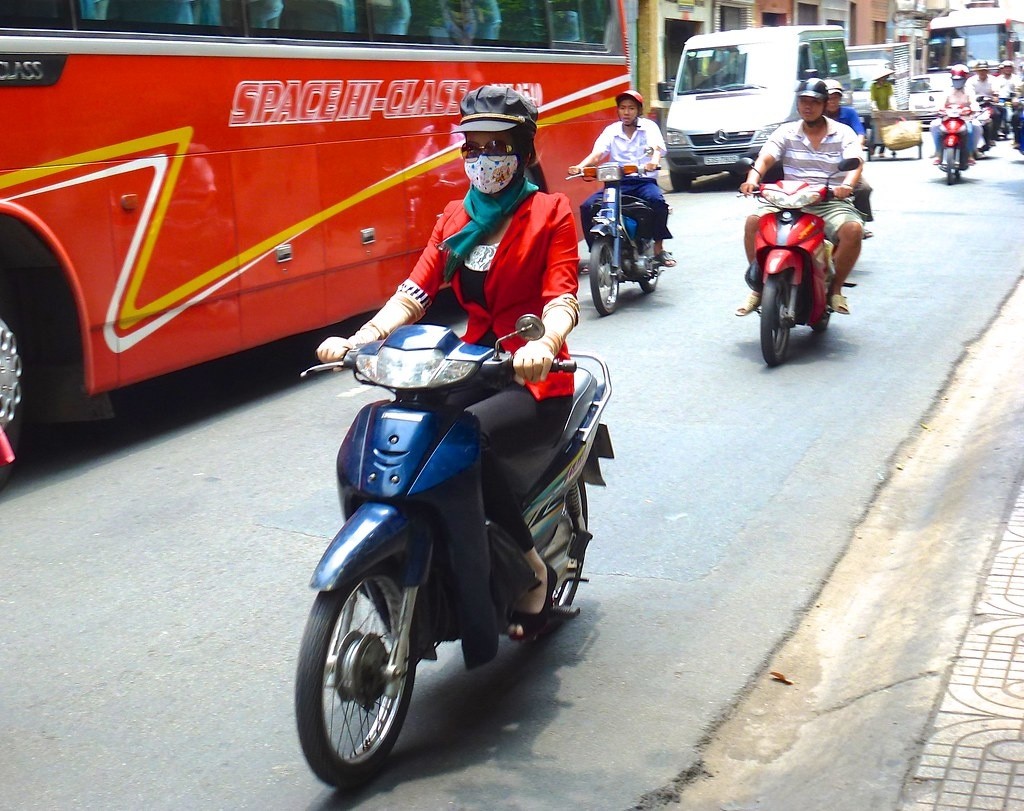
[564,147,674,317]
[929,93,1024,185]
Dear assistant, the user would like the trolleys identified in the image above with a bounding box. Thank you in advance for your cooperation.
[858,110,923,162]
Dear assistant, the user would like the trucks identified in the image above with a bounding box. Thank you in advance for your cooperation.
[845,41,914,130]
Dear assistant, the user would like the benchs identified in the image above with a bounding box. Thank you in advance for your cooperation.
[79,1,580,43]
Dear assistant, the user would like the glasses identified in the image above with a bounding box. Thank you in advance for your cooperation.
[461,139,520,162]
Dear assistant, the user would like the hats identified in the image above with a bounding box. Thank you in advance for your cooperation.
[872,67,895,82]
[449,85,538,139]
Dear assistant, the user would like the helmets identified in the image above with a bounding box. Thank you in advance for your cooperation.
[616,89,642,107]
[999,61,1014,69]
[824,78,843,96]
[975,61,988,73]
[951,64,969,80]
[796,77,828,101]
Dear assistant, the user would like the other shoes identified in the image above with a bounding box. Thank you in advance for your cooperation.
[969,158,976,164]
[990,140,995,146]
[934,160,940,165]
[1014,143,1020,148]
[510,564,558,641]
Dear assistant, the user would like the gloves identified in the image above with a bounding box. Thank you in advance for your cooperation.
[511,304,576,388]
[317,294,424,374]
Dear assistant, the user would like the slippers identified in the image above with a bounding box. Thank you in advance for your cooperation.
[653,250,675,267]
[828,294,849,314]
[736,294,761,316]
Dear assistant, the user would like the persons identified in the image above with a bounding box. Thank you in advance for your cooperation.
[568,90,677,275]
[735,78,862,316]
[315,86,579,641]
[823,79,875,240]
[871,68,897,158]
[930,59,1024,165]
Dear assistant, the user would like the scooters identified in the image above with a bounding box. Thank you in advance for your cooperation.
[292,313,616,792]
[732,155,862,368]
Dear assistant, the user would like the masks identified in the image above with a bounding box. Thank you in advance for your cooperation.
[464,154,521,194]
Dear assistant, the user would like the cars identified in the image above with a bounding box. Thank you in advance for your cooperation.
[907,72,954,131]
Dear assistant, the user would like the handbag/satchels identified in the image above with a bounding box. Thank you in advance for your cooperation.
[882,116,923,149]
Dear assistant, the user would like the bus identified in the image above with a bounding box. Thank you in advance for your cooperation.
[912,7,1024,77]
[0,1,635,492]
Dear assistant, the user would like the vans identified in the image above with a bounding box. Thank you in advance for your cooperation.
[655,24,853,192]
